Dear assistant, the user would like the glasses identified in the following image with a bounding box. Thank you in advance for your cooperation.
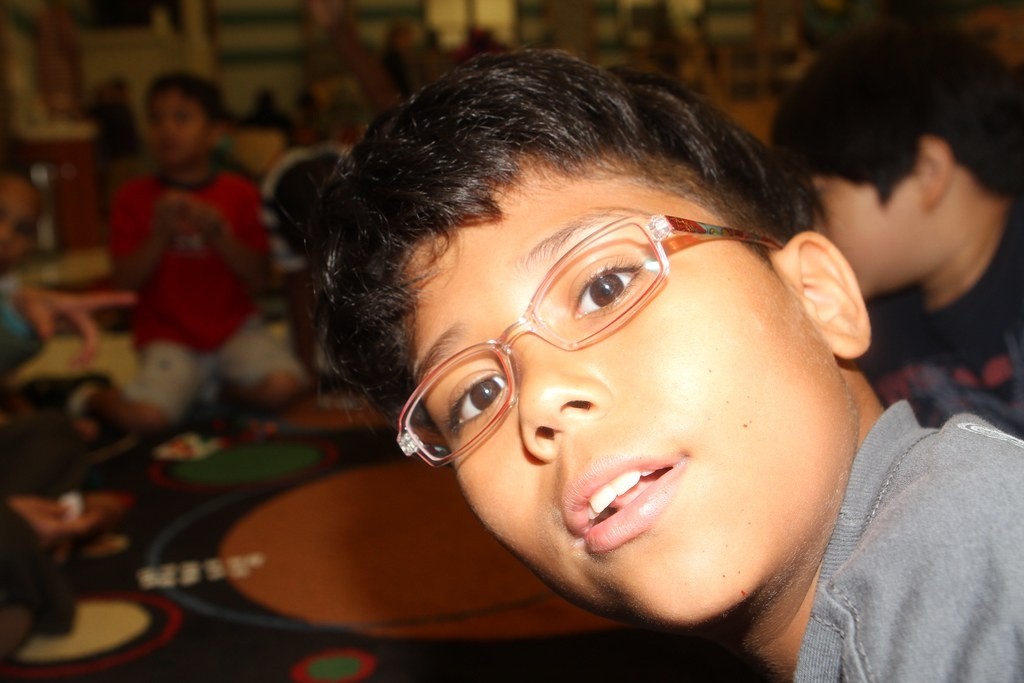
[395,212,785,469]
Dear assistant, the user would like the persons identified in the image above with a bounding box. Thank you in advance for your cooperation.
[311,0,1024,682]
[0,69,330,683]
